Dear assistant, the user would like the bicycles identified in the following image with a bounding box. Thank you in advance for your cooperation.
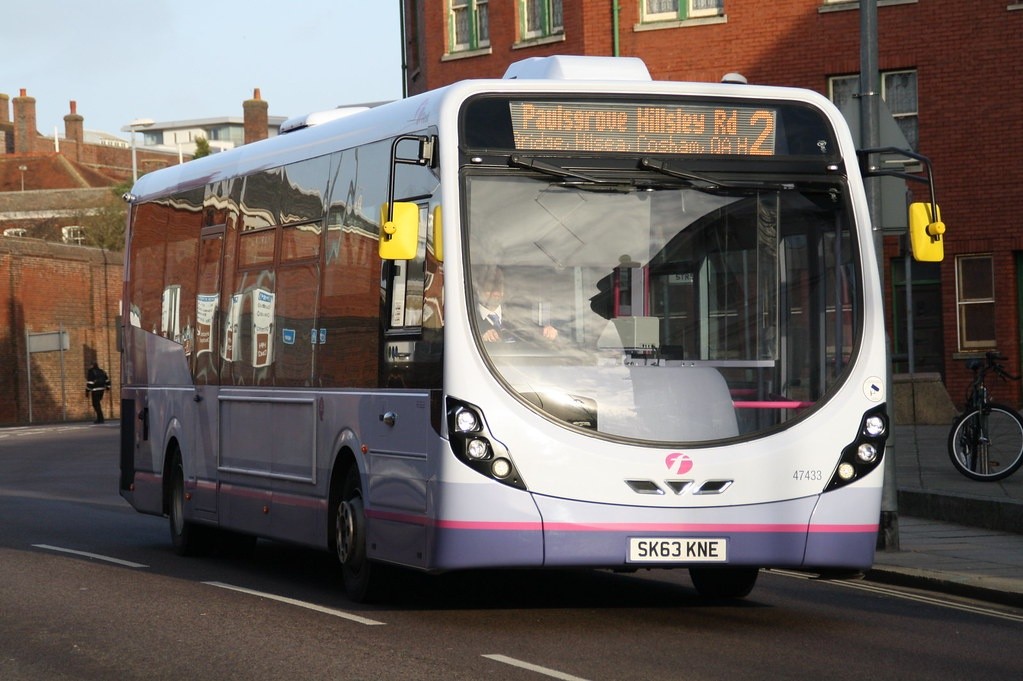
[946,350,1023,483]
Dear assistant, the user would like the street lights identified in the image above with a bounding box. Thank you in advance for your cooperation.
[18,164,27,191]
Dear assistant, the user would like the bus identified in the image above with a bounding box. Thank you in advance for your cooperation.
[117,53,946,600]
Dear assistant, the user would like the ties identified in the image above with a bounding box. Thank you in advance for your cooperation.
[489,314,501,328]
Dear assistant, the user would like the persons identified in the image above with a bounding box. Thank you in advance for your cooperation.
[473,266,558,343]
[85,362,111,424]
